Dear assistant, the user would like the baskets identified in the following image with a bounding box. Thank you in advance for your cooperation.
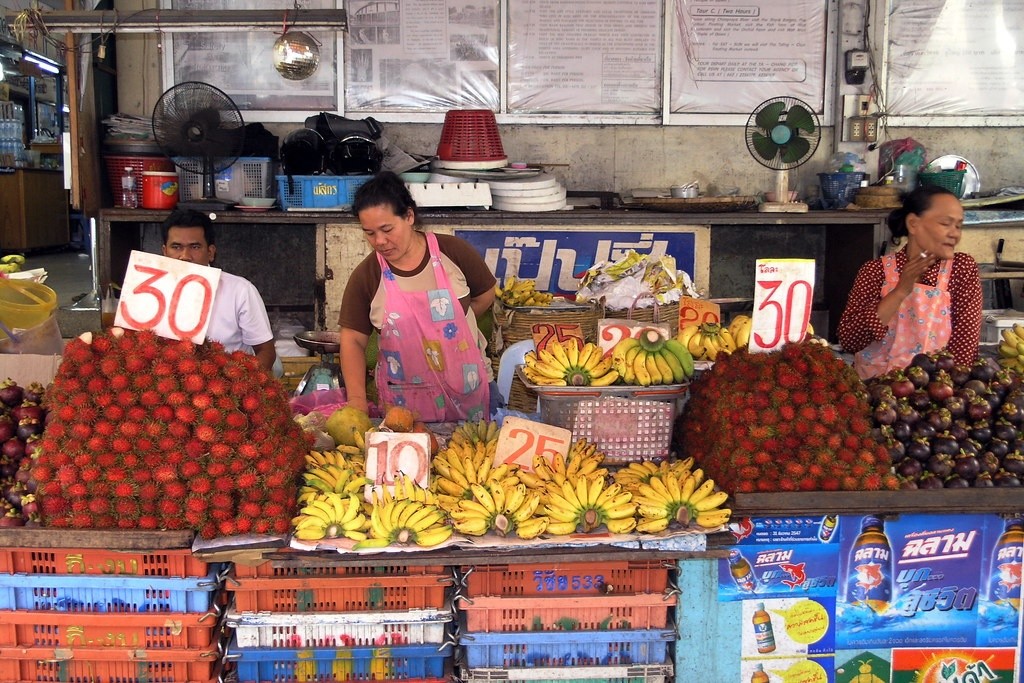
[486,301,681,413]
[916,168,966,197]
[435,110,509,162]
[104,149,374,210]
[0,545,679,683]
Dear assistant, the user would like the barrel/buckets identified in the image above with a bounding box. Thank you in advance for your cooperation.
[0,272,65,356]
[142,171,179,210]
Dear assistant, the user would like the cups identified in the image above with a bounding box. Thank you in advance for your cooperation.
[886,165,916,191]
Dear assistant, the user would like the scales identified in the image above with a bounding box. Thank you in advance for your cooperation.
[291,330,340,397]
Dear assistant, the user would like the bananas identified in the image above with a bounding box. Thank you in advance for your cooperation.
[995,322,1024,378]
[493,275,554,307]
[521,313,815,388]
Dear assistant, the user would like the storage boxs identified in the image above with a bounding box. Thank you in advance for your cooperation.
[978,307,1024,343]
[274,174,375,211]
[1,547,680,683]
[170,154,273,204]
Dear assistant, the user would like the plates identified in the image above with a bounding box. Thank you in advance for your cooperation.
[234,205,276,213]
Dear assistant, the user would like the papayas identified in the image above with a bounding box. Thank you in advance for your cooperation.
[293,418,735,549]
[325,407,371,446]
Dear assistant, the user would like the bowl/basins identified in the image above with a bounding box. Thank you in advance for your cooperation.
[764,191,798,202]
[670,188,699,199]
[239,198,276,207]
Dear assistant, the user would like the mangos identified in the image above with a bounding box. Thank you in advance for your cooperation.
[383,406,438,452]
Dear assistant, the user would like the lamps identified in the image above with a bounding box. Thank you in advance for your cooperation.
[22,49,66,75]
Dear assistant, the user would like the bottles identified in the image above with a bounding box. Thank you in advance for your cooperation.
[727,549,757,592]
[752,603,776,653]
[845,515,894,614]
[122,167,138,209]
[988,516,1024,612]
[750,663,769,683]
[0,101,24,167]
[818,514,838,542]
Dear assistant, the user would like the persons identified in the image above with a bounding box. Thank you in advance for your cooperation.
[838,185,983,371]
[336,171,497,421]
[161,210,277,372]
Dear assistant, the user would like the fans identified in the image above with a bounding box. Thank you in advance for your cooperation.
[151,81,246,211]
[745,96,821,212]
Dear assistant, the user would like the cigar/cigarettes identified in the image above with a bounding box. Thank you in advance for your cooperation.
[920,251,929,260]
